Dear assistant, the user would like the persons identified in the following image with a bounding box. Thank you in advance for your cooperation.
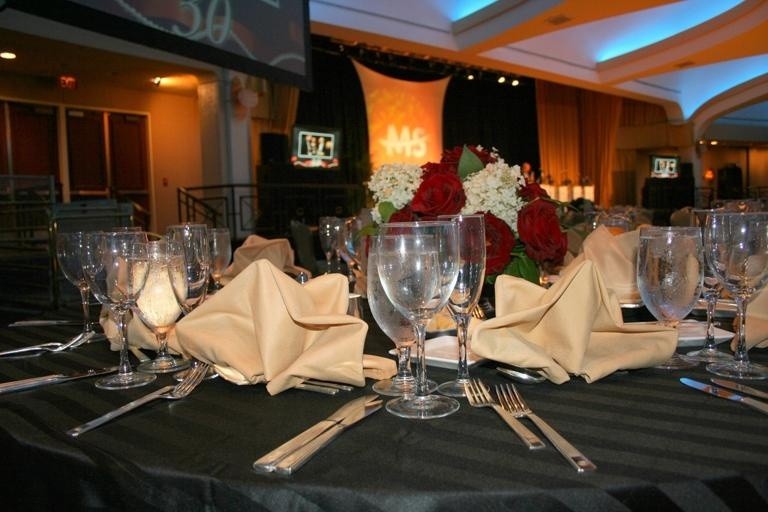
[654,158,677,178]
[297,135,332,160]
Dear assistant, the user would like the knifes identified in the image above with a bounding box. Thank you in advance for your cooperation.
[678,377,768,414]
[0,364,133,397]
[252,396,384,478]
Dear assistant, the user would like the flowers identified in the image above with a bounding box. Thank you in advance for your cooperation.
[350,144,594,288]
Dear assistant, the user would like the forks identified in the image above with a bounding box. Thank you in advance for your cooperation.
[65,361,210,438]
[0,331,95,361]
[459,376,598,480]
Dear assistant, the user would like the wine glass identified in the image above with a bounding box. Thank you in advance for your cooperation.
[637,213,767,382]
[365,214,490,421]
[52,222,233,390]
[320,217,362,283]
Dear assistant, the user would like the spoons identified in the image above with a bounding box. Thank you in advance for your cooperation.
[495,366,629,382]
